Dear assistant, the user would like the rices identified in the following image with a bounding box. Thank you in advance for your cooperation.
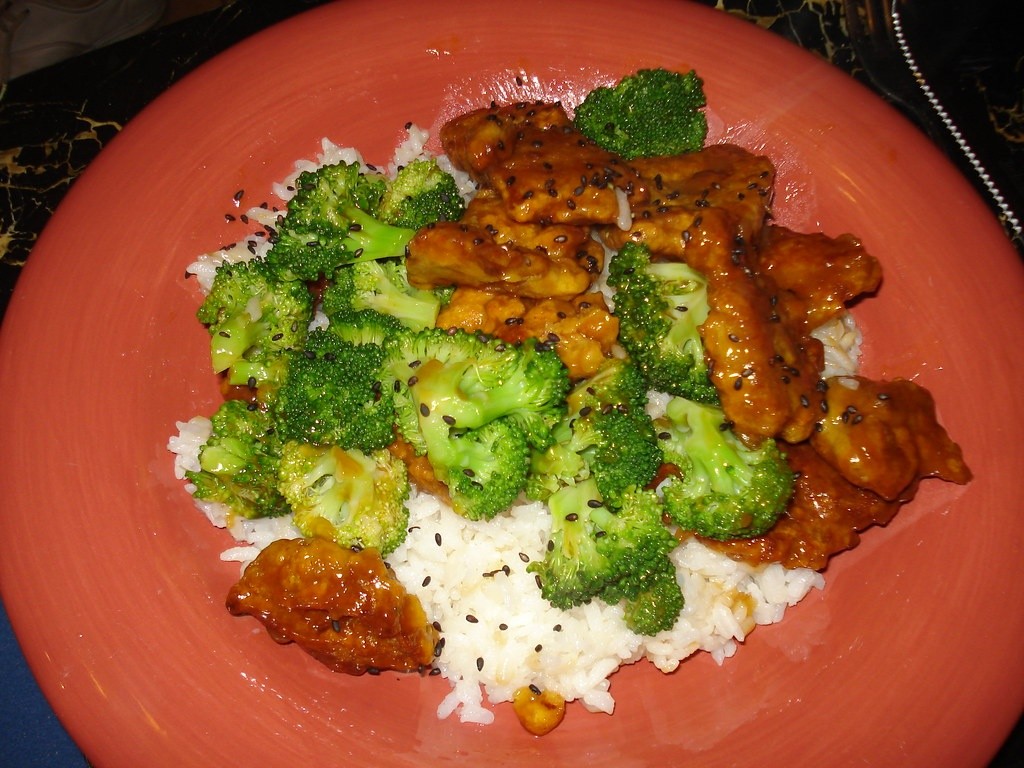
[168,123,867,727]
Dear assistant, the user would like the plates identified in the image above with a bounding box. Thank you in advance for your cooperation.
[1,1,1023,768]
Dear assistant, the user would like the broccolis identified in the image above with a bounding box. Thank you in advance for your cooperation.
[185,68,800,637]
